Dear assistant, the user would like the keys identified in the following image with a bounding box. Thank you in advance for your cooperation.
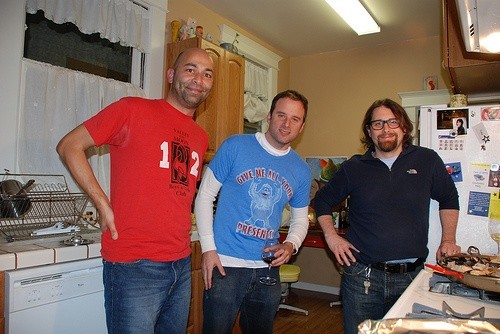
[364,281,370,294]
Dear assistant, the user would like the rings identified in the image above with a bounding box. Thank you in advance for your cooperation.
[284,259,286,262]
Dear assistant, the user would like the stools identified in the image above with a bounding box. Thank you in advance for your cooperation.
[277,263,309,316]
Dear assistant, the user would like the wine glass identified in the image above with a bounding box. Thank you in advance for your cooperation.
[488,213,500,257]
[260,238,281,286]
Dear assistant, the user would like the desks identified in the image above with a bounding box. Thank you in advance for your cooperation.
[278,225,347,248]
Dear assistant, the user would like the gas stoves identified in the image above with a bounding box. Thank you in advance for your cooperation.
[392,273,500,319]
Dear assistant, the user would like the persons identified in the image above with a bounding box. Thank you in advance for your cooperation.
[56,47,215,334]
[315,98,461,334]
[195,90,312,334]
[456,119,465,135]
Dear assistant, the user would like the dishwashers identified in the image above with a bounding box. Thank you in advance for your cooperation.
[4,258,109,334]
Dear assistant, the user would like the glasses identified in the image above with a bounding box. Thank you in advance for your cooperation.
[368,118,401,130]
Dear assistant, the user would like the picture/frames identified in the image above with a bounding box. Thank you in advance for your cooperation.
[304,156,348,209]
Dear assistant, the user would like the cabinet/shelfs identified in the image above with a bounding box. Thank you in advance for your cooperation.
[441,0,500,96]
[167,36,246,164]
[186,240,204,334]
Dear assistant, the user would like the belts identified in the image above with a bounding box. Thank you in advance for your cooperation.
[356,257,426,274]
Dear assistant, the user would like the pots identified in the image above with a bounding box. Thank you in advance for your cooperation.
[423,252,500,293]
[0,179,36,219]
